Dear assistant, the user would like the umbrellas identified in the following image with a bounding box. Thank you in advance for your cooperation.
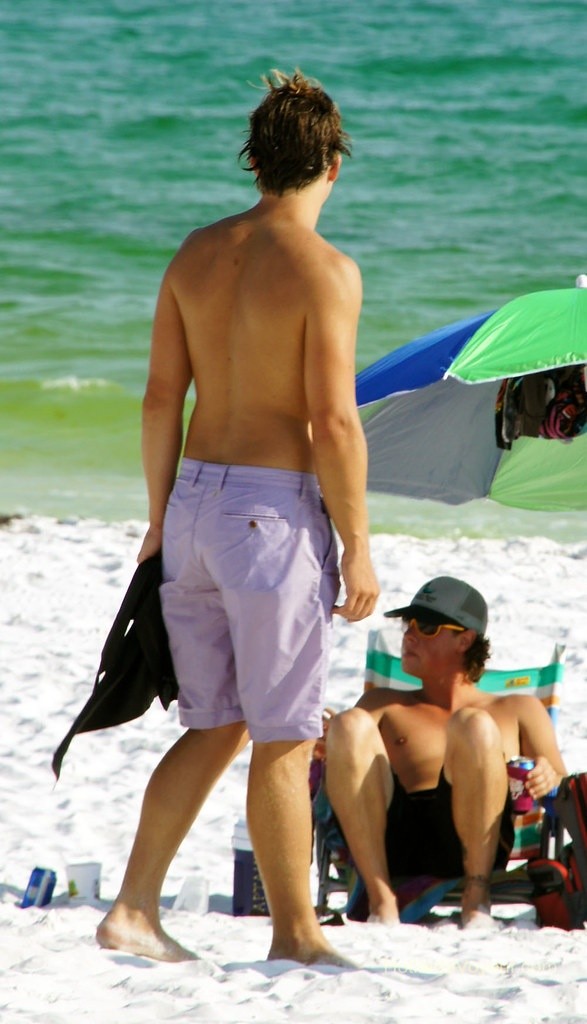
[356,274,587,514]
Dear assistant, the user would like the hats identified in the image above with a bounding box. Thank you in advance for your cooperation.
[383,576,488,634]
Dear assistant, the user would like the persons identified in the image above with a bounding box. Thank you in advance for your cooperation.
[314,576,568,928]
[98,75,380,971]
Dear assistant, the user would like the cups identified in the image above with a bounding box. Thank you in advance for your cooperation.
[65,865,101,906]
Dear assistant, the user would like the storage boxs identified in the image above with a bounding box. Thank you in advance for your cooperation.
[230,821,271,915]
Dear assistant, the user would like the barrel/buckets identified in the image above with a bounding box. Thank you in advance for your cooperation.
[231,819,272,917]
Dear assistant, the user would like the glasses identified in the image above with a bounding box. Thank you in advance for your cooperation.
[403,614,468,639]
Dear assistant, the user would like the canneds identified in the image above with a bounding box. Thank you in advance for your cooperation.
[507,756,535,771]
[20,866,57,909]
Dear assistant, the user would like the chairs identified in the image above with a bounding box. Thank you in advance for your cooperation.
[308,625,567,926]
